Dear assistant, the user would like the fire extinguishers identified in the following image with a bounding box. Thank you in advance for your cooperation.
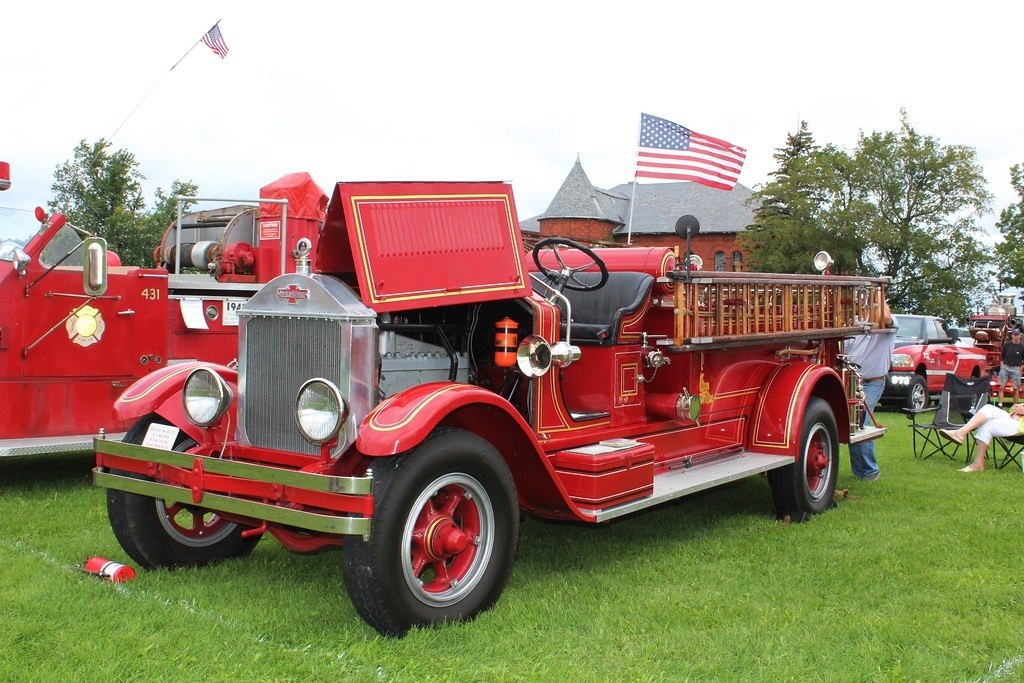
[74,556,136,584]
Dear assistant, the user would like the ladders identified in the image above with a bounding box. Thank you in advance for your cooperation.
[655,269,897,352]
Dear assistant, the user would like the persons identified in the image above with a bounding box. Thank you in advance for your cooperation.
[842,285,900,485]
[997,328,1024,407]
[938,402,1024,473]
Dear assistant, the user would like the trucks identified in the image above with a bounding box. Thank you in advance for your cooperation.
[0,160,1023,636]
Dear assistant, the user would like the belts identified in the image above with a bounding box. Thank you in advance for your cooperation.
[861,378,882,383]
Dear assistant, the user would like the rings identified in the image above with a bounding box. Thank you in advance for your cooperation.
[1015,410,1016,412]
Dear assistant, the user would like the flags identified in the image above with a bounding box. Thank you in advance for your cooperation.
[200,22,230,61]
[632,112,749,192]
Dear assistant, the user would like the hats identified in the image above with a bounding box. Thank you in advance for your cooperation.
[1012,329,1021,335]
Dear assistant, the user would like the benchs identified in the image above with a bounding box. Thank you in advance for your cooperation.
[529,271,655,346]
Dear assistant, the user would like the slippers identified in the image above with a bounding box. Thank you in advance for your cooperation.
[956,464,983,472]
[939,428,964,446]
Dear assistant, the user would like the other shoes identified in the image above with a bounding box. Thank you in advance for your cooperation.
[996,404,1003,409]
[861,473,880,483]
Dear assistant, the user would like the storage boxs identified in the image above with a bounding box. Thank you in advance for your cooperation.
[555,444,656,510]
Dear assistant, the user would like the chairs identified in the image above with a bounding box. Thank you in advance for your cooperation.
[902,372,991,464]
[993,435,1024,469]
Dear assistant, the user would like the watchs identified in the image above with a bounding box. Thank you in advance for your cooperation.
[877,286,881,291]
